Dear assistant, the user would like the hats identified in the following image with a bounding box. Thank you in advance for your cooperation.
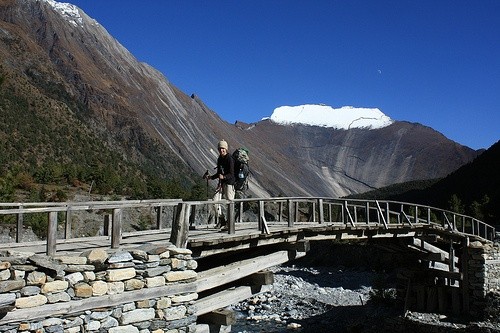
[217,140,228,152]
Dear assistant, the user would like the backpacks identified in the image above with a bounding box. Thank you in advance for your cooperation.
[230,145,249,205]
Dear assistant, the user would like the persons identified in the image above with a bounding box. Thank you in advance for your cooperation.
[204,140,235,233]
